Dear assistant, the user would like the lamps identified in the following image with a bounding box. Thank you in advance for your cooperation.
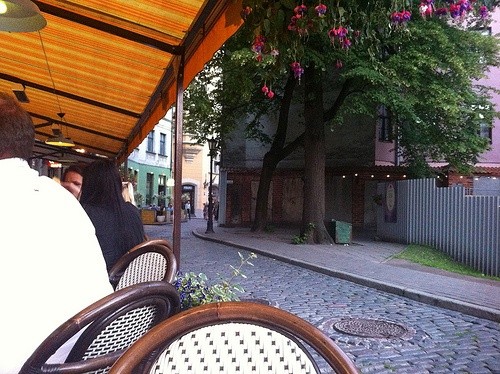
[0,0,47,33]
[44,113,76,147]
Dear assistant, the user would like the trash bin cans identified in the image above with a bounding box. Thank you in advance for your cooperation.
[326,219,353,244]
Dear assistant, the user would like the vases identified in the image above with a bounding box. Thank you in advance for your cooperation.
[157,216,166,222]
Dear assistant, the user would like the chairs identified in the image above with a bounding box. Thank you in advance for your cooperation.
[17,280,181,374]
[107,301,365,374]
[108,239,176,283]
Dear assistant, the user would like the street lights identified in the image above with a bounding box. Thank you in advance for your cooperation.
[205,135,217,234]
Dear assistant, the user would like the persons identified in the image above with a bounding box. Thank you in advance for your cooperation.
[78,159,145,292]
[60,165,84,203]
[205,202,219,220]
[138,203,174,221]
[184,201,191,221]
[0,90,114,374]
